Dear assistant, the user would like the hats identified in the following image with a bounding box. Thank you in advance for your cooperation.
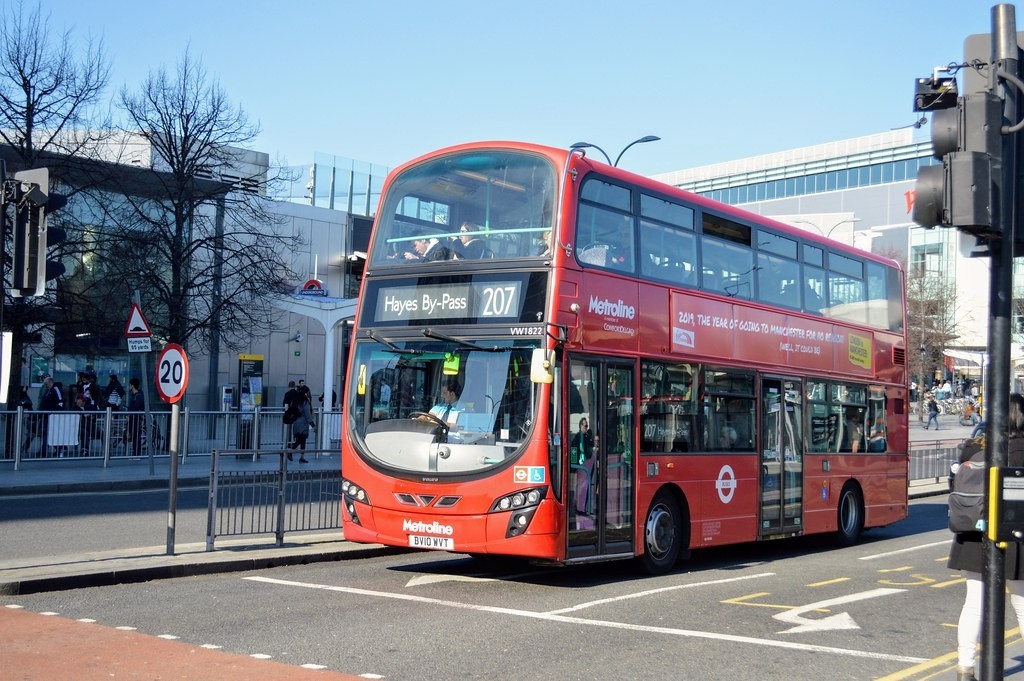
[77,394,87,400]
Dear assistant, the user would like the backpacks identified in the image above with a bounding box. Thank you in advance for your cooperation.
[948,449,985,533]
[283,396,312,424]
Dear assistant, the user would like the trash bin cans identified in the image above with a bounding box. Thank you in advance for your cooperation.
[219,384,233,414]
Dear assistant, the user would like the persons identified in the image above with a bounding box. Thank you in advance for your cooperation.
[923,397,938,430]
[720,427,737,448]
[319,390,337,411]
[911,379,959,402]
[673,429,689,453]
[404,221,487,261]
[833,406,871,449]
[948,394,1024,681]
[418,380,474,432]
[537,231,551,257]
[284,379,316,463]
[380,378,415,418]
[20,357,145,457]
[572,417,599,466]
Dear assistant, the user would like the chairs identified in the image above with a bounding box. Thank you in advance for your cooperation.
[479,235,851,313]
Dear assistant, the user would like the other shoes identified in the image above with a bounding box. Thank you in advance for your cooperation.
[81,450,89,456]
[923,426,928,429]
[287,453,293,461]
[35,451,48,457]
[299,458,308,463]
[934,428,938,430]
[52,452,62,456]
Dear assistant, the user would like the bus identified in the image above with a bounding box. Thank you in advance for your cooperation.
[336,138,912,578]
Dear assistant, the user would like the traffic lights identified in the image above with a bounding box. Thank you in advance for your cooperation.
[14,167,68,297]
[912,150,995,233]
[929,91,1003,165]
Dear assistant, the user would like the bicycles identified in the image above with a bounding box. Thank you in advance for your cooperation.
[913,396,980,416]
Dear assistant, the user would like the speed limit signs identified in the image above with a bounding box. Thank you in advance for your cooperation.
[155,343,189,405]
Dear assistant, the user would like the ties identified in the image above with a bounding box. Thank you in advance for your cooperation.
[441,405,452,423]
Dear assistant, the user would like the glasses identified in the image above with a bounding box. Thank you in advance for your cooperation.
[78,399,87,402]
